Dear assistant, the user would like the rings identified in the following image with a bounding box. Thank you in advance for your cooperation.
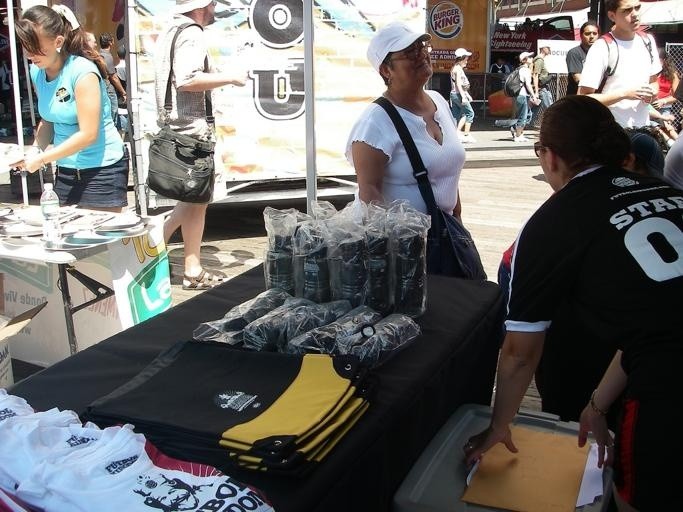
[605,442,616,449]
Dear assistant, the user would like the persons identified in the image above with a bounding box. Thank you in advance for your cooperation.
[443,0,683,149]
[145,0,248,292]
[497,126,668,427]
[8,3,130,214]
[346,25,487,277]
[463,92,678,507]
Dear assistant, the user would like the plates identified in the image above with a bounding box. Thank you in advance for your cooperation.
[0,208,143,250]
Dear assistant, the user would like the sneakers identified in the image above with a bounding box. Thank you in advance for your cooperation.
[460,126,532,144]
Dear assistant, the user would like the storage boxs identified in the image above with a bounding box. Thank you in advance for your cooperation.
[395,402,615,512]
[0,273,47,391]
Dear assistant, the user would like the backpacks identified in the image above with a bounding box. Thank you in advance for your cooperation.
[504,66,531,97]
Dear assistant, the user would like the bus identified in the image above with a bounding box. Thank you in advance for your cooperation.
[489,10,578,69]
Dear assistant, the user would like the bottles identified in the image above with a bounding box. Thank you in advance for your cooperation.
[641,86,652,105]
[41,182,64,241]
[218,223,428,365]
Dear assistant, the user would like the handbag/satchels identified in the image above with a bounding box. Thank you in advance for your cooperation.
[143,128,218,209]
[428,209,486,279]
[460,82,471,92]
[533,68,551,87]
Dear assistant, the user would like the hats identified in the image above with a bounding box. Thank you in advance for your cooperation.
[366,20,433,76]
[454,46,473,58]
[175,0,231,16]
[628,130,667,176]
[518,43,554,63]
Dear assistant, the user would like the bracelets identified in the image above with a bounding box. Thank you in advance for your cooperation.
[586,387,613,420]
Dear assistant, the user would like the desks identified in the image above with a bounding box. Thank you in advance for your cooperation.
[0,202,165,355]
[6,260,508,512]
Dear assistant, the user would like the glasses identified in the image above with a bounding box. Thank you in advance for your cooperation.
[530,137,552,161]
[384,43,433,63]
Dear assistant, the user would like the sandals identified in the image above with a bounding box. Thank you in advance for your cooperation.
[181,269,229,291]
